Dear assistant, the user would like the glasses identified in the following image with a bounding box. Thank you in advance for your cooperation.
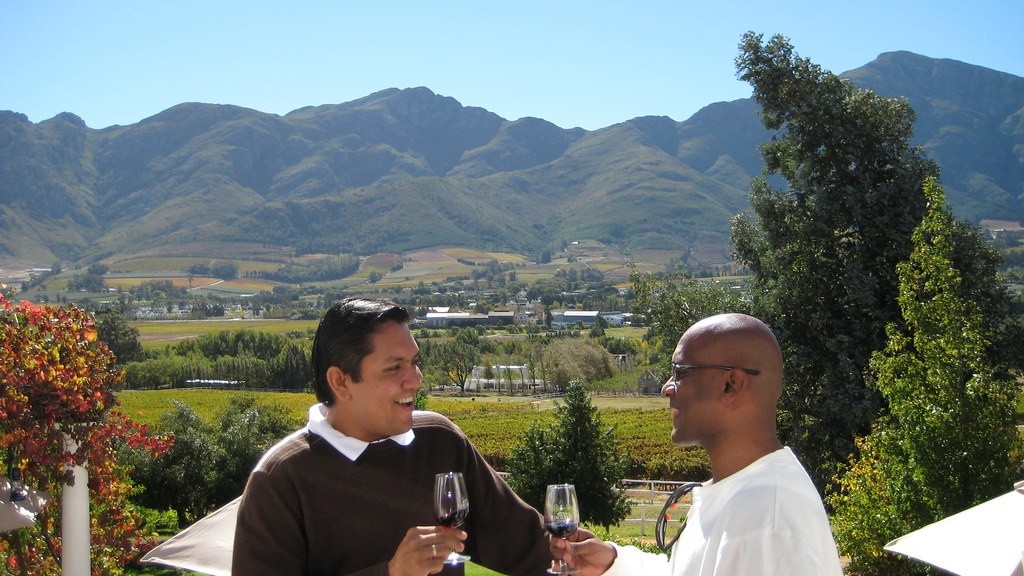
[671,362,760,385]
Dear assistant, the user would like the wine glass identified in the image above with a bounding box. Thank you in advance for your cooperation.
[433,472,470,564]
[545,484,581,574]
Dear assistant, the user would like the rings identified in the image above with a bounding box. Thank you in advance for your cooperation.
[432,544,437,558]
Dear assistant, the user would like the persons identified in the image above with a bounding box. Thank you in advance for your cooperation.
[549,313,845,576]
[231,296,557,576]
